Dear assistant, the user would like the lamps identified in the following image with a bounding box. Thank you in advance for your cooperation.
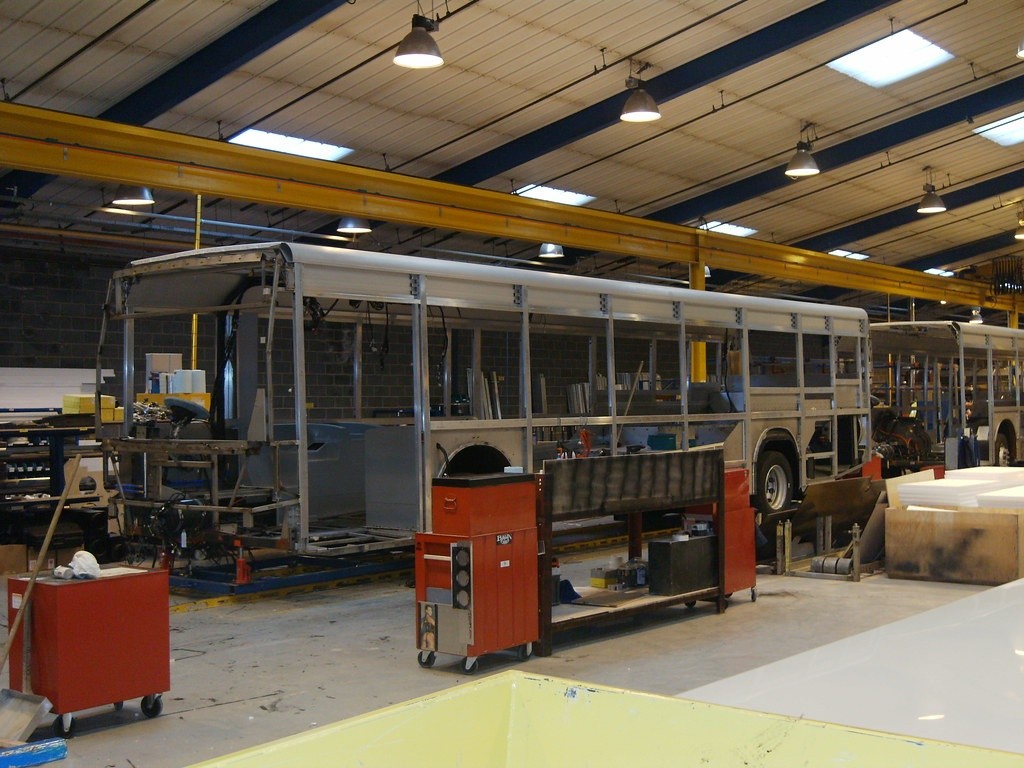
[620,76,662,122]
[112,184,156,206]
[784,142,820,177]
[917,184,946,214]
[539,244,563,258]
[337,217,373,234]
[1014,219,1024,240]
[392,13,444,68]
[969,307,983,325]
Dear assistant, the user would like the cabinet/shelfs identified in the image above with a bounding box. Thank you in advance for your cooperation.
[0,407,119,513]
[684,467,757,595]
[647,534,719,596]
[415,472,540,656]
[7,562,170,714]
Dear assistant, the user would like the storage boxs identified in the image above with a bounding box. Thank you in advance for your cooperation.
[0,688,54,749]
[137,393,210,412]
[0,542,84,576]
[590,568,617,588]
[62,393,124,423]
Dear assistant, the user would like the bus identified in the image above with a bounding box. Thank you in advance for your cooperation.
[837,319,1024,480]
[118,242,872,586]
